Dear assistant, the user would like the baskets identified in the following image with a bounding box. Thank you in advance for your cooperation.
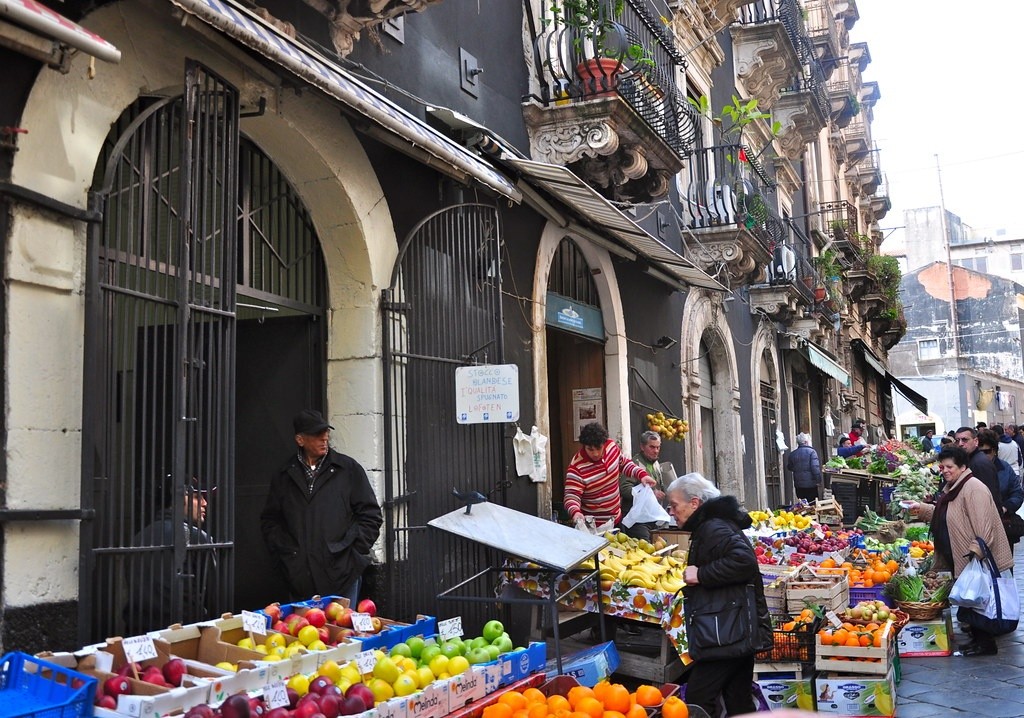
[895,590,948,620]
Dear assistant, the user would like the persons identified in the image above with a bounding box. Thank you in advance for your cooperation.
[922,430,934,454]
[837,419,872,458]
[666,473,775,718]
[621,430,668,541]
[564,423,656,532]
[125,474,217,632]
[788,432,821,504]
[941,430,956,447]
[955,427,1003,637]
[977,431,1024,514]
[976,422,986,431]
[261,409,383,610]
[992,424,1024,484]
[901,446,1014,658]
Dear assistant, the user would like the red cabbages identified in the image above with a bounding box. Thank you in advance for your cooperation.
[875,450,900,472]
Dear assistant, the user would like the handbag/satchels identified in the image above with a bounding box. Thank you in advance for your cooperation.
[999,512,1024,544]
[681,582,763,660]
[956,536,1020,635]
[948,557,991,610]
[622,483,671,527]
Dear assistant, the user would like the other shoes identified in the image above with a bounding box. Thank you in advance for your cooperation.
[958,642,978,649]
[960,623,972,632]
[963,647,997,656]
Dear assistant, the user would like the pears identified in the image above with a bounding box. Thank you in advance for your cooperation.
[603,530,689,560]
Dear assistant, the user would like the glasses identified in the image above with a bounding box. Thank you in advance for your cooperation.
[980,447,993,455]
[953,437,973,446]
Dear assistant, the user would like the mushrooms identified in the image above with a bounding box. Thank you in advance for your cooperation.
[922,571,946,590]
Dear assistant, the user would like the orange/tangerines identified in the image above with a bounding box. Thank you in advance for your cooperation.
[481,676,689,718]
[754,608,895,663]
[815,549,899,587]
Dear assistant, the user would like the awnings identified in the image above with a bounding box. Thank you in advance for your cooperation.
[863,351,928,415]
[169,0,522,205]
[0,0,121,74]
[803,341,849,386]
[493,155,733,295]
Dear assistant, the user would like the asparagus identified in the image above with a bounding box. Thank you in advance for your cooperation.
[929,578,957,603]
[880,573,925,603]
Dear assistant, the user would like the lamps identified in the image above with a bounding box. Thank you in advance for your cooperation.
[652,335,678,355]
[474,257,504,279]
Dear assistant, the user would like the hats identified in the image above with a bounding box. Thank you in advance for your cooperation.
[851,423,864,431]
[154,470,218,512]
[924,430,932,436]
[293,410,328,434]
[839,437,850,444]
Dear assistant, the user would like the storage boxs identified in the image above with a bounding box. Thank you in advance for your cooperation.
[822,474,890,525]
[746,568,953,718]
[0,594,682,718]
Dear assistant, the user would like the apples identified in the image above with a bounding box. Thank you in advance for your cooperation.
[61,599,526,718]
[646,411,689,442]
[747,509,862,566]
[846,600,897,622]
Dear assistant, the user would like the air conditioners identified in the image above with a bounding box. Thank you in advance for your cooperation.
[765,247,797,282]
[694,184,738,226]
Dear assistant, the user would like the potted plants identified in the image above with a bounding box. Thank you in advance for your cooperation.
[832,220,848,240]
[538,0,656,100]
[802,250,844,308]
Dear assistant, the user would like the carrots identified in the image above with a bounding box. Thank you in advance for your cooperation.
[912,540,936,551]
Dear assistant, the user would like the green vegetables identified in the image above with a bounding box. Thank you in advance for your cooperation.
[823,455,890,475]
[904,436,924,449]
[897,449,917,466]
[904,525,932,540]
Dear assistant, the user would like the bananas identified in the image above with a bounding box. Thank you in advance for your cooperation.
[574,545,688,593]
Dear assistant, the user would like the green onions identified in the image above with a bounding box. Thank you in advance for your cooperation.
[855,505,904,541]
